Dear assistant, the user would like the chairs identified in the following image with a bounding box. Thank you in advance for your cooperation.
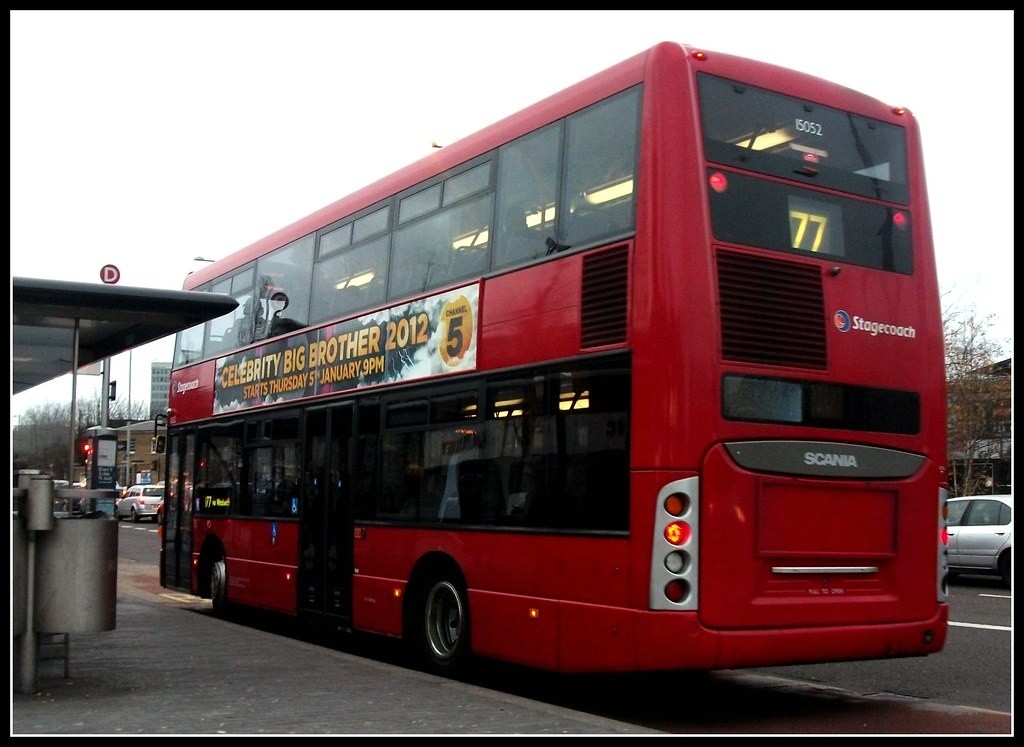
[202,208,619,357]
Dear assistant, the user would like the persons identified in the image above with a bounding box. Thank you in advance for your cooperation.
[501,207,533,262]
[232,297,270,347]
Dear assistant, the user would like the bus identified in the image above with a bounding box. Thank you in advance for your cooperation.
[152,42,953,679]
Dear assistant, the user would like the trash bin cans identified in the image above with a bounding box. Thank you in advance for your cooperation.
[34,487,121,636]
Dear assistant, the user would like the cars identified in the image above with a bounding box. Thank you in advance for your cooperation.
[51,477,124,513]
[116,484,165,524]
[157,488,193,523]
[945,495,1011,588]
